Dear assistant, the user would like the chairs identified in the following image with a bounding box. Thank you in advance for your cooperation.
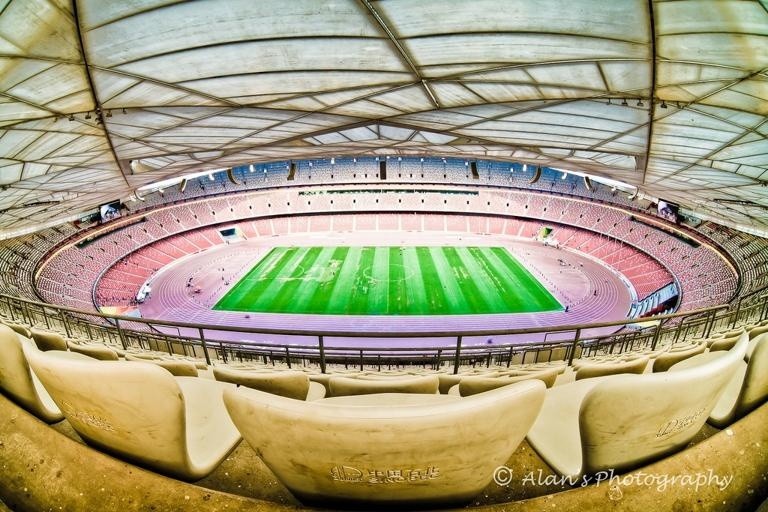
[0,314,768,510]
